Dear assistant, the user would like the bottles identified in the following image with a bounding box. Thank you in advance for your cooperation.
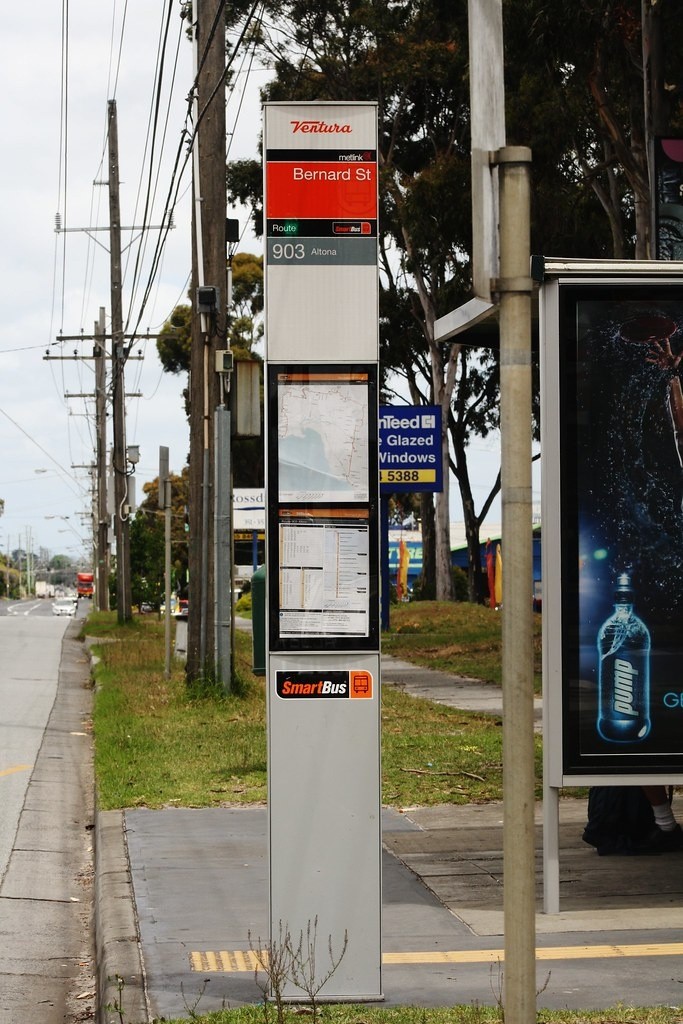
[597,575,650,742]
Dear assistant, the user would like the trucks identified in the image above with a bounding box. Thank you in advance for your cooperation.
[77,573,94,600]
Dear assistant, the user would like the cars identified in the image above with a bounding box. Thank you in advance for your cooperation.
[52,598,77,618]
[141,592,188,614]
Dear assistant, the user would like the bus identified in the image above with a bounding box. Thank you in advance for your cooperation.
[34,570,78,599]
